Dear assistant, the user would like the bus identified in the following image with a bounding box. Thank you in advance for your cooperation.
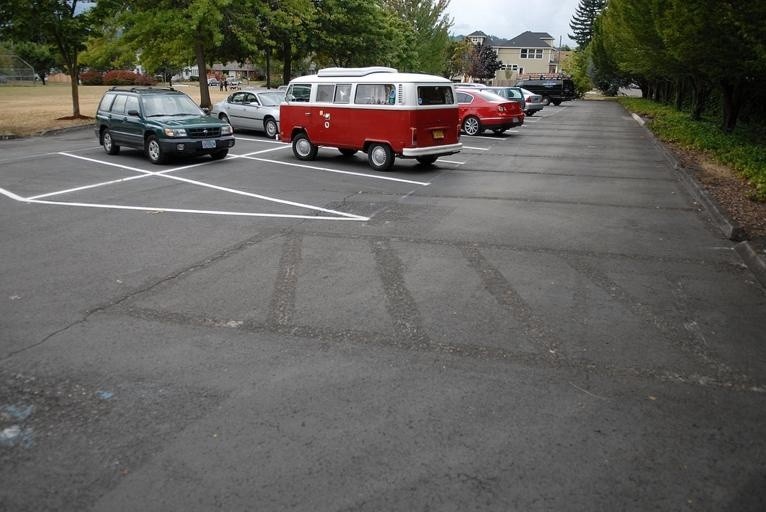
[279,66,463,172]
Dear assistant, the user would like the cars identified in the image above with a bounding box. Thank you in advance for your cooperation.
[277,85,311,102]
[208,75,240,86]
[212,89,285,139]
[454,82,544,137]
[93,84,235,165]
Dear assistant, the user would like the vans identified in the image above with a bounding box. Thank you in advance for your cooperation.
[514,75,575,106]
